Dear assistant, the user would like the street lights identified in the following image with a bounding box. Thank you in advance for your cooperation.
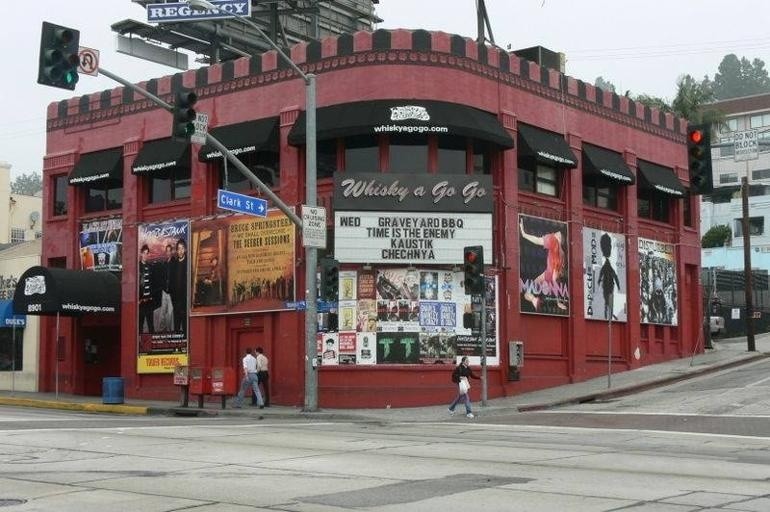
[186,0,318,411]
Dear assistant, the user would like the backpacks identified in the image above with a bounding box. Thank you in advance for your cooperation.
[452,368,459,383]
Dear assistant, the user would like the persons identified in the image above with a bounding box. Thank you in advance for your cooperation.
[231,348,264,409]
[518,218,569,312]
[169,238,187,334]
[138,244,157,334]
[449,356,485,417]
[157,245,175,332]
[249,344,271,407]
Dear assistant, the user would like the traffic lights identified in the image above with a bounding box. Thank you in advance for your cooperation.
[37,21,80,90]
[686,123,714,196]
[464,246,485,295]
[171,85,197,144]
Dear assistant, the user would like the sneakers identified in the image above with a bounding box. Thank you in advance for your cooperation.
[448,408,455,415]
[467,413,474,418]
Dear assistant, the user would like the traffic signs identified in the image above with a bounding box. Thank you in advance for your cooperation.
[216,187,268,218]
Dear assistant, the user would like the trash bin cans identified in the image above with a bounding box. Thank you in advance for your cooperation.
[102,377,123,404]
